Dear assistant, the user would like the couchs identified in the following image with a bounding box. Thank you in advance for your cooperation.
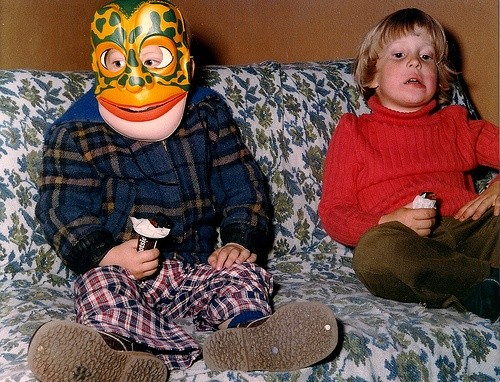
[0,57,500,382]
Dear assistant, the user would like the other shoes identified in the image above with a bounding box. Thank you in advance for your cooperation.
[27,320,167,382]
[464,270,500,323]
[204,300,339,371]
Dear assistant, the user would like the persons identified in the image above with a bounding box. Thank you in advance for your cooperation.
[318,7,499,322]
[27,0,339,382]
